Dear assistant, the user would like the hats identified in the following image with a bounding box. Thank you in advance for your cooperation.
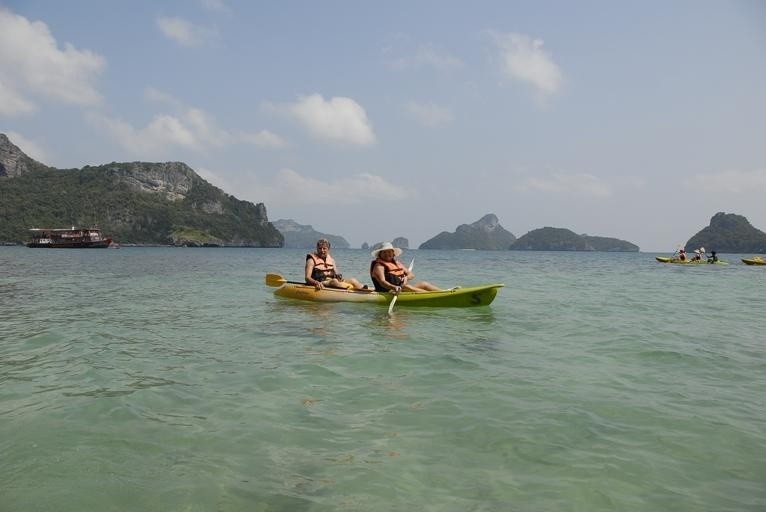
[693,246,705,253]
[370,242,402,258]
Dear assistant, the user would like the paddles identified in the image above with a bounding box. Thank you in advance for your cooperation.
[388,256,415,314]
[265,273,353,290]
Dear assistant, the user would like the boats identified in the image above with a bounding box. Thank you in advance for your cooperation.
[270,282,505,309]
[655,257,733,265]
[23,224,110,251]
[740,256,765,264]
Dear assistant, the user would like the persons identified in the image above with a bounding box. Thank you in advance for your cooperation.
[706,249,719,265]
[691,249,703,261]
[671,248,687,260]
[304,236,368,292]
[369,239,442,294]
